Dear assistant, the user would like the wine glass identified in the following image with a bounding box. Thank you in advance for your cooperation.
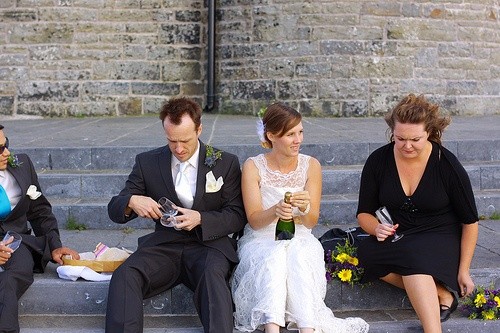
[159,214,184,231]
[375,206,404,242]
[0,231,22,272]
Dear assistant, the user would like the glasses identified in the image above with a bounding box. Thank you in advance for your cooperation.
[0,136,10,154]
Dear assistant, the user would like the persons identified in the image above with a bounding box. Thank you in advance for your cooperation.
[102,97,248,333]
[231,103,369,332]
[356,95,478,332]
[0,126,63,333]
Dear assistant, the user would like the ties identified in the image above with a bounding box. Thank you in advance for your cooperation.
[174,161,193,210]
[0,184,12,218]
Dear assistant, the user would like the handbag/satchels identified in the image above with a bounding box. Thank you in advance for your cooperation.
[318,225,376,283]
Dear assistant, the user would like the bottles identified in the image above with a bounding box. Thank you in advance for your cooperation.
[275,192,296,240]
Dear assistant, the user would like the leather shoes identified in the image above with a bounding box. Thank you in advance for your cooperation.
[440,292,458,322]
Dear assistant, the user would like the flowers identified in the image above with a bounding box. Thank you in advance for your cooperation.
[8,149,23,168]
[204,145,224,168]
[326,238,372,288]
[459,282,500,320]
[256,107,272,150]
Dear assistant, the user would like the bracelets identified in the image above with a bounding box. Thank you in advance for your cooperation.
[296,201,312,217]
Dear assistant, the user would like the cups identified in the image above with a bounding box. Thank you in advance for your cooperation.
[158,197,179,216]
